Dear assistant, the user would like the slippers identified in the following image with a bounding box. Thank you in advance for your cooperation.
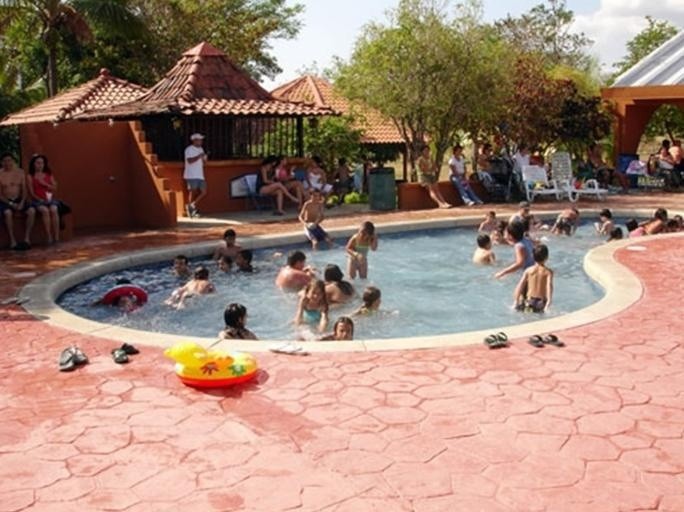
[111,343,139,364]
[530,335,543,347]
[542,334,563,346]
[497,332,508,346]
[485,334,497,347]
[60,345,87,371]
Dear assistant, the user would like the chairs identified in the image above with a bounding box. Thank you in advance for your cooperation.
[647,154,681,191]
[228,174,276,216]
[619,154,649,194]
[523,153,609,203]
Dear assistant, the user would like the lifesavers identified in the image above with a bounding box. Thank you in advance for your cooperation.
[164,343,257,387]
[97,284,148,305]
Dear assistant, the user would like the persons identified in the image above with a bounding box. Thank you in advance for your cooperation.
[114,133,382,339]
[416,139,684,312]
[26,154,63,247]
[1,153,35,251]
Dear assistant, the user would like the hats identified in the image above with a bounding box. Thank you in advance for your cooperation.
[519,201,530,209]
[191,134,204,140]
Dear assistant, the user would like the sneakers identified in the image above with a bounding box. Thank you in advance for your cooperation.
[186,203,201,216]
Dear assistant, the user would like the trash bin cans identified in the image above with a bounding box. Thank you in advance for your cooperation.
[369,168,396,210]
[618,153,639,189]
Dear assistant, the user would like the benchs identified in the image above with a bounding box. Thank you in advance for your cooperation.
[0,213,61,251]
